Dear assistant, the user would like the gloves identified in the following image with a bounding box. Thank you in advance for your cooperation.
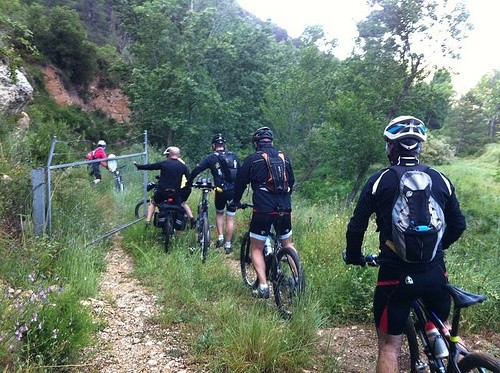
[229,199,242,208]
[345,255,365,267]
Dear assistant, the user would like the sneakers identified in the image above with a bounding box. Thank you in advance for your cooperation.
[292,277,300,287]
[252,285,269,300]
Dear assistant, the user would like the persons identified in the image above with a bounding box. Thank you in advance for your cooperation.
[187,134,241,254]
[134,146,195,229]
[347,116,474,373]
[229,127,300,298]
[90,140,109,186]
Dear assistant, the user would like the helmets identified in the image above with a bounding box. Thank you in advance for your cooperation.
[253,126,274,143]
[168,146,180,159]
[97,140,106,147]
[383,115,426,143]
[211,134,227,144]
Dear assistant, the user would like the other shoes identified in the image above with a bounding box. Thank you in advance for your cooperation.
[215,238,224,248]
[144,221,149,228]
[190,219,197,229]
[223,243,231,254]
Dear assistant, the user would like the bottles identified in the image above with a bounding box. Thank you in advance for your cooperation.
[264,237,274,257]
[424,320,450,359]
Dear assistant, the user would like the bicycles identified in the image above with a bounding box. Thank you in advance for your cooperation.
[134,182,187,254]
[112,169,123,193]
[347,254,500,373]
[229,203,306,321]
[187,181,216,265]
